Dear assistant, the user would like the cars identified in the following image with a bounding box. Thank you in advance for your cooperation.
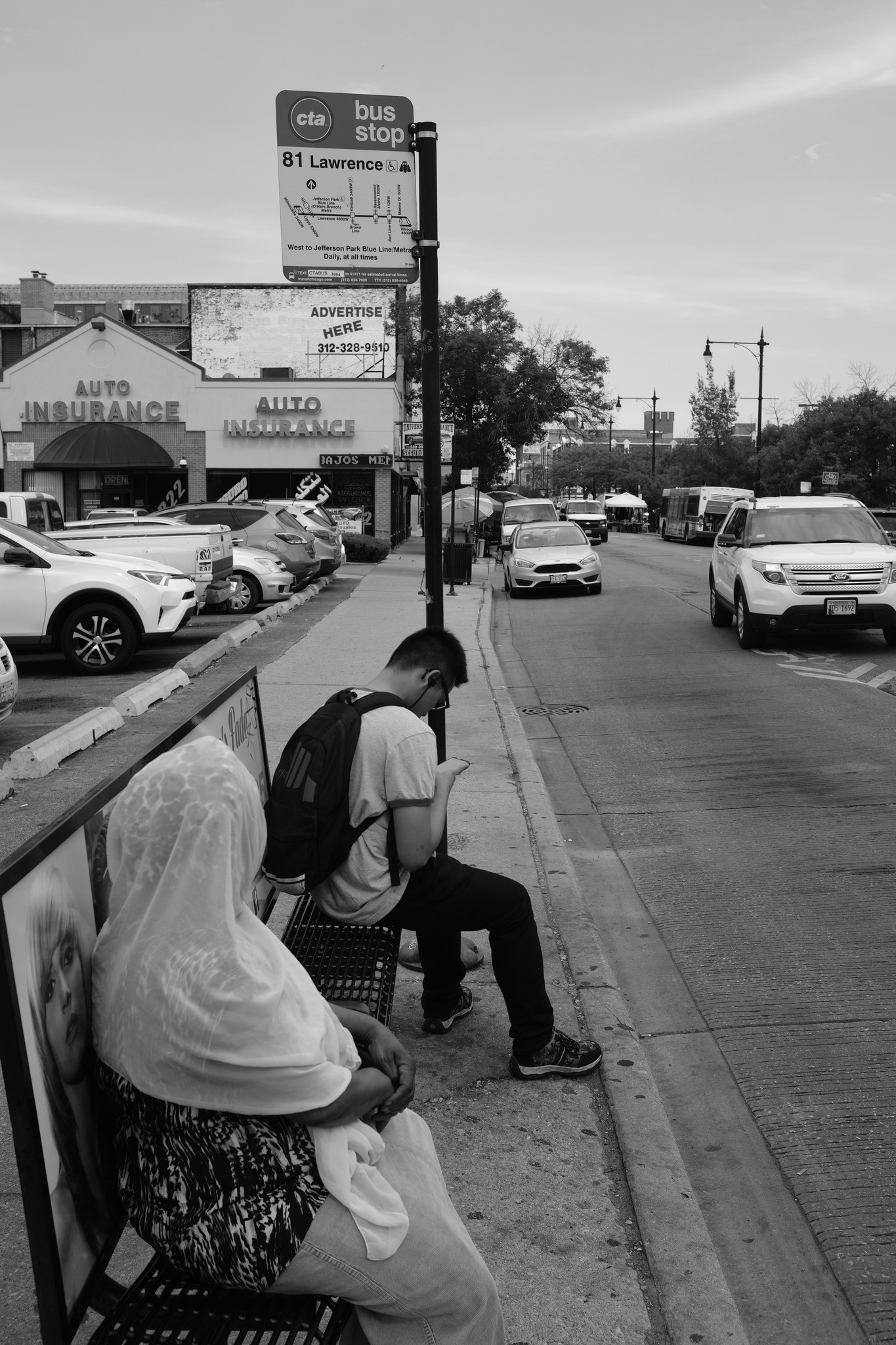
[62,517,298,614]
[340,508,362,521]
[407,429,451,445]
[499,521,602,599]
[0,635,19,724]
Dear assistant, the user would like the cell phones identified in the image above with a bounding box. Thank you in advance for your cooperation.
[437,757,471,772]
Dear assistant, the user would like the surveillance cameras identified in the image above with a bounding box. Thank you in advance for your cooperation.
[180,459,187,468]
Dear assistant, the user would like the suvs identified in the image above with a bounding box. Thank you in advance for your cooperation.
[86,508,149,520]
[867,507,896,546]
[146,501,322,583]
[559,499,609,543]
[236,499,347,579]
[0,516,200,676]
[708,492,896,650]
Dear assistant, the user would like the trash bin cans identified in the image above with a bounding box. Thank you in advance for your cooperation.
[442,542,475,582]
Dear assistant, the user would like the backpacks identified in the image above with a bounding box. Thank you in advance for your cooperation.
[260,688,407,896]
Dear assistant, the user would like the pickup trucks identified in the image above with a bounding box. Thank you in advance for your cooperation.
[0,491,234,608]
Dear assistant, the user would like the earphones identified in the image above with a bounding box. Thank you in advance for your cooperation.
[428,677,437,687]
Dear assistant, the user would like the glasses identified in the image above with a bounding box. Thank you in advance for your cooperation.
[421,668,449,711]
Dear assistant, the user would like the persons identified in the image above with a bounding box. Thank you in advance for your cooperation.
[418,507,425,537]
[91,738,505,1345]
[309,626,603,1076]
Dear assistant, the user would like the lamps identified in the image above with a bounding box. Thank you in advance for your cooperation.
[179,456,188,468]
[91,318,106,331]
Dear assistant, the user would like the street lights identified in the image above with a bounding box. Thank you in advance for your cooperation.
[528,452,550,499]
[703,326,770,498]
[616,387,660,533]
[547,439,578,500]
[580,413,615,529]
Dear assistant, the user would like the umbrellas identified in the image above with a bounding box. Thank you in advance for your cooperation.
[484,491,529,508]
[441,494,494,527]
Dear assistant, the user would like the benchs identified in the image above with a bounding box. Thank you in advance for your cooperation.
[88,877,401,1345]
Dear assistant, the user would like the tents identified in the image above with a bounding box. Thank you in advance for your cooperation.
[605,492,648,527]
[442,486,503,552]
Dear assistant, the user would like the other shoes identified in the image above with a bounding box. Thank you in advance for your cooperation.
[421,536,424,537]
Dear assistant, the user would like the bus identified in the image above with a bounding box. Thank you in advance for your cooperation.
[659,486,755,545]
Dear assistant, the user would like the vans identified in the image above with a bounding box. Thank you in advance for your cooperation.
[494,498,567,557]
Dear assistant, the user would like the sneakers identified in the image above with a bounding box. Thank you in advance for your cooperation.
[421,986,474,1033]
[509,1026,603,1080]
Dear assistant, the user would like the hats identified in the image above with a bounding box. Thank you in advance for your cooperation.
[420,507,423,510]
[611,513,615,516]
